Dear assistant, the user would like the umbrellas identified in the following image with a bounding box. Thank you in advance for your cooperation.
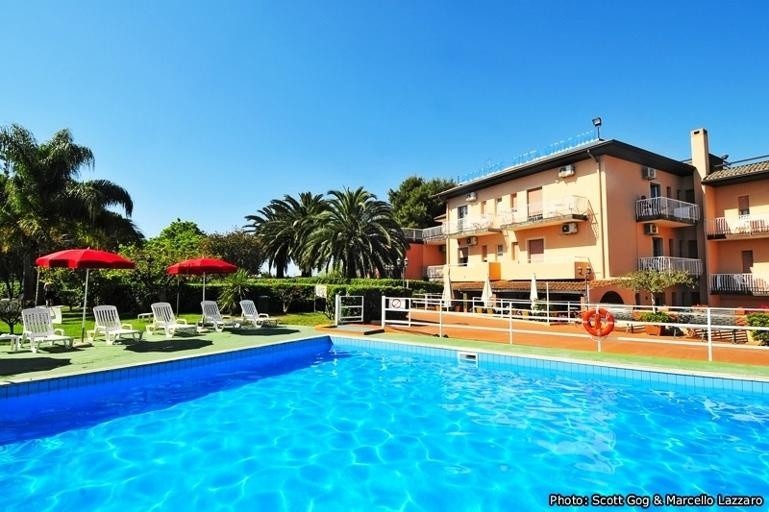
[480,274,493,309]
[529,272,539,310]
[34,246,137,342]
[166,256,239,329]
[439,265,454,312]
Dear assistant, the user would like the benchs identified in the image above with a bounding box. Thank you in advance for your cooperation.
[137,310,152,323]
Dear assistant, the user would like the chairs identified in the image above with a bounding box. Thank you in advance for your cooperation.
[677,302,750,345]
[236,297,282,330]
[145,300,199,339]
[732,270,745,288]
[638,199,657,216]
[430,297,535,321]
[641,257,668,273]
[17,304,74,353]
[199,299,240,334]
[85,304,145,348]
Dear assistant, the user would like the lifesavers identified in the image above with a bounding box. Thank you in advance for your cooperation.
[584,309,613,335]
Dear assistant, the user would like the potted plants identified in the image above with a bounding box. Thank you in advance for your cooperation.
[639,310,674,334]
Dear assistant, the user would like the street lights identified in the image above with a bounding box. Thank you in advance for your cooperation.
[396,255,409,288]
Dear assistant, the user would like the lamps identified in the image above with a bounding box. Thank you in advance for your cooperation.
[592,116,605,145]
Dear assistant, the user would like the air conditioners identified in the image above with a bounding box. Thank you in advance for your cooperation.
[557,164,577,178]
[643,166,655,180]
[644,222,661,236]
[465,190,478,202]
[561,221,580,234]
[465,235,478,246]
